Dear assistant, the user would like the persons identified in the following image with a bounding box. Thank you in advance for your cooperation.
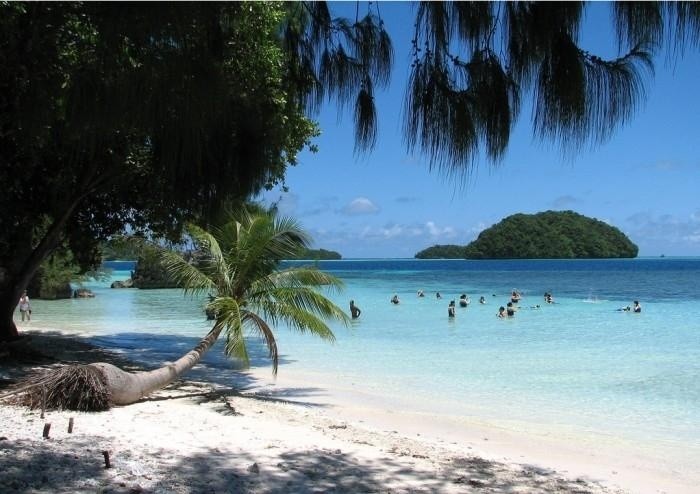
[621,300,642,313]
[417,289,424,297]
[19,290,31,321]
[391,296,399,304]
[436,292,441,299]
[496,288,522,318]
[544,292,553,304]
[350,300,361,319]
[448,294,471,317]
[479,296,486,304]
